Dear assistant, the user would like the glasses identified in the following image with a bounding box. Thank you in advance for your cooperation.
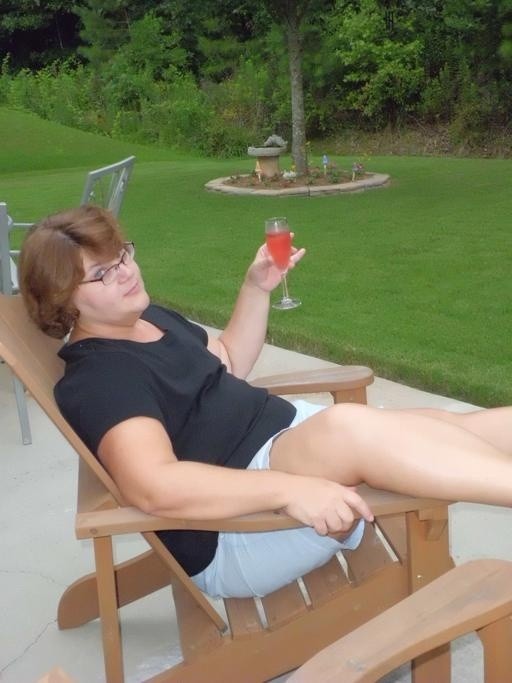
[79,242,135,285]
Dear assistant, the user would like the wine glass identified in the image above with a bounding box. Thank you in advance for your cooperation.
[264,216,303,311]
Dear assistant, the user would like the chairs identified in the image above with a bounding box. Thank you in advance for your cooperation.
[0,155,137,445]
[284,558,511,683]
[0,295,512,683]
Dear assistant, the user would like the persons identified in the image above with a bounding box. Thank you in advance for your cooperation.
[19,205,512,599]
